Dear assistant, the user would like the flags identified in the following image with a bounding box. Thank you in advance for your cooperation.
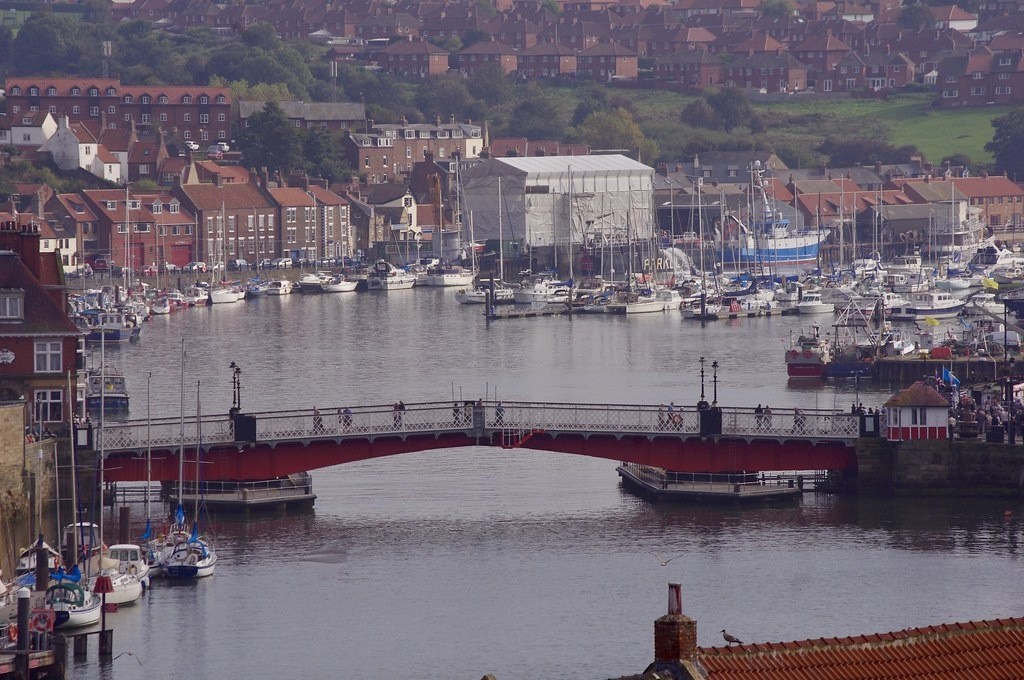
[926,315,939,326]
[936,372,946,388]
[943,367,960,384]
[984,277,999,290]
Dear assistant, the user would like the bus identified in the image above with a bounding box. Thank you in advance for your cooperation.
[62,264,93,280]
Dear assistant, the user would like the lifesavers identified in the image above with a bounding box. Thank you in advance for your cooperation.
[33,614,51,631]
[790,349,799,359]
[188,554,198,565]
[680,301,687,310]
[158,533,163,543]
[8,623,18,642]
[673,415,683,424]
[803,350,812,359]
[128,564,137,575]
[54,556,60,572]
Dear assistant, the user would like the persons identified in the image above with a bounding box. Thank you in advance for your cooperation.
[756,404,805,434]
[493,401,505,428]
[452,403,463,427]
[313,406,352,436]
[858,399,1024,435]
[477,398,483,406]
[658,401,685,431]
[393,400,407,430]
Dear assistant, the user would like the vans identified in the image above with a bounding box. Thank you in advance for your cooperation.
[351,254,364,264]
[227,259,250,271]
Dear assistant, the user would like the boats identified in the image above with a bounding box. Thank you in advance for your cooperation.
[87,363,132,412]
[64,185,444,324]
[614,460,803,498]
[782,323,831,378]
[86,311,133,344]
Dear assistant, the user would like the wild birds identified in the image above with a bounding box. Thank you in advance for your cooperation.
[719,629,744,646]
[647,550,684,566]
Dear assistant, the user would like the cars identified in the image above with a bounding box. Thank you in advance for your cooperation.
[250,258,271,271]
[207,145,222,154]
[208,153,224,160]
[183,261,206,274]
[205,261,225,273]
[159,264,182,275]
[185,140,200,151]
[270,257,293,270]
[217,142,230,152]
[135,265,158,277]
[111,266,132,277]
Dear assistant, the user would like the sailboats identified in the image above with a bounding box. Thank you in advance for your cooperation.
[0,368,191,639]
[452,160,1024,358]
[428,190,477,288]
[160,378,223,582]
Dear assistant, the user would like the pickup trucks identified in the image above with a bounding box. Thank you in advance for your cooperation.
[94,258,108,273]
